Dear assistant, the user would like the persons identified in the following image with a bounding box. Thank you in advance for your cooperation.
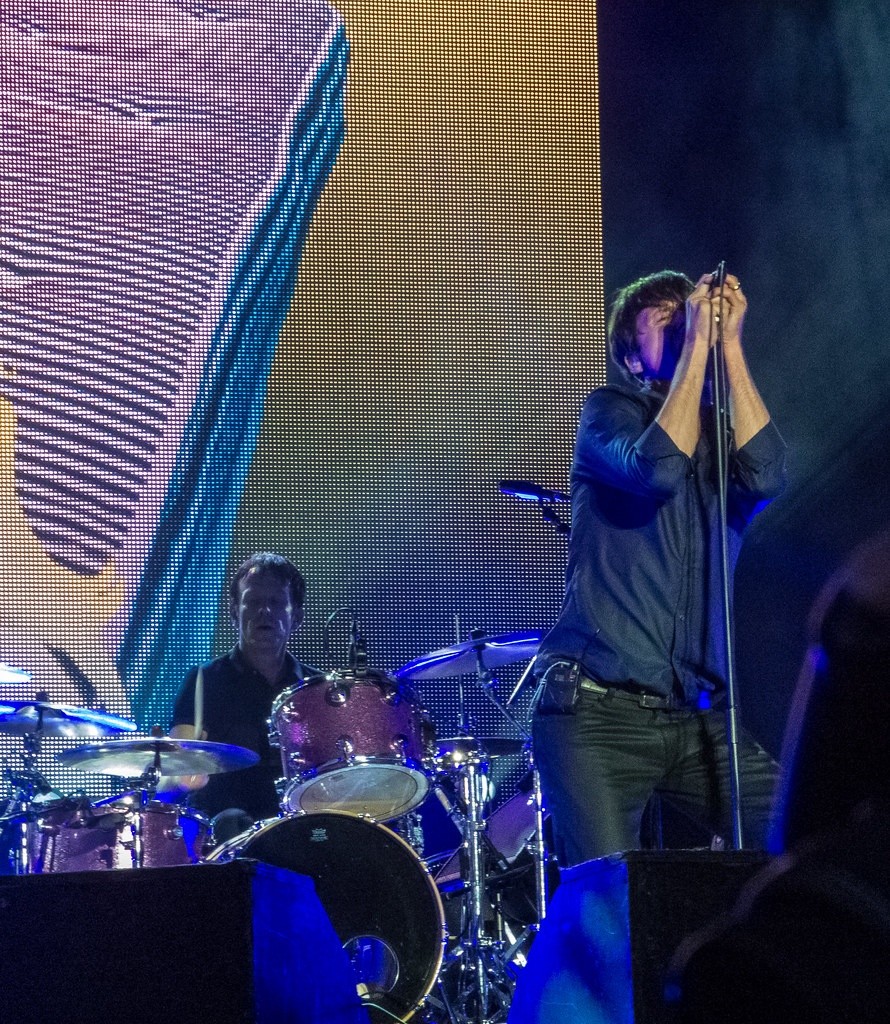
[530,270,799,872]
[171,551,330,863]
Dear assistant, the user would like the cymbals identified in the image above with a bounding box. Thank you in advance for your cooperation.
[438,735,528,761]
[56,736,261,777]
[0,698,137,739]
[395,629,545,681]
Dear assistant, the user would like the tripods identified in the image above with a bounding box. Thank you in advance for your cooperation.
[409,747,548,1024]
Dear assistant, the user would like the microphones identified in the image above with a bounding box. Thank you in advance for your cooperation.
[68,813,125,829]
[507,655,539,708]
[709,261,728,291]
[348,619,361,670]
[499,480,571,502]
[19,753,53,795]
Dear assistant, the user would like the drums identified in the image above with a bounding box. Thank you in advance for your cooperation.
[201,809,446,1024]
[21,797,190,873]
[271,669,432,823]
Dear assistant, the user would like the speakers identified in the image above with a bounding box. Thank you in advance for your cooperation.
[0,859,372,1024]
[506,848,769,1024]
[479,788,560,919]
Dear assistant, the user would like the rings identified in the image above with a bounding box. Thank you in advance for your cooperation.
[731,283,743,293]
